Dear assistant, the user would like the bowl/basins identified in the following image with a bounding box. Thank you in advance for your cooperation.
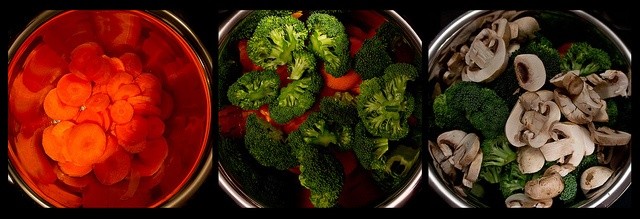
[428,10,632,210]
[7,9,212,209]
[217,9,422,208]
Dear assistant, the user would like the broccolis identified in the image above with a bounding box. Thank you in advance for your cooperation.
[431,36,618,200]
[218,11,423,209]
